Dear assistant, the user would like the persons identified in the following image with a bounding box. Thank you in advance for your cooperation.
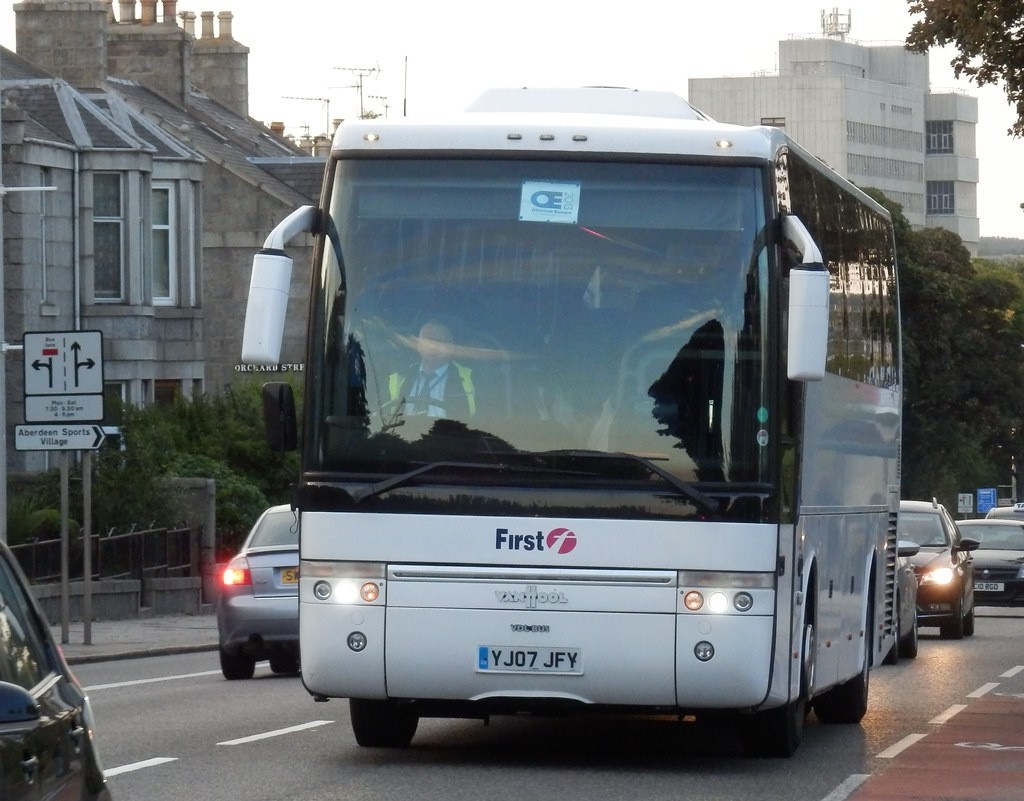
[379,320,479,429]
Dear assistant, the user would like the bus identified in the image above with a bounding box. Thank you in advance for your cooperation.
[239,108,900,762]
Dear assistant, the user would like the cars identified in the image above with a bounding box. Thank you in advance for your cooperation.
[218,504,302,678]
[985,504,1024,523]
[956,519,1024,608]
[900,496,980,639]
[0,540,113,800]
[883,539,922,667]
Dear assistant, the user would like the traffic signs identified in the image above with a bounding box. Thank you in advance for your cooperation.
[13,330,107,451]
[977,488,997,513]
